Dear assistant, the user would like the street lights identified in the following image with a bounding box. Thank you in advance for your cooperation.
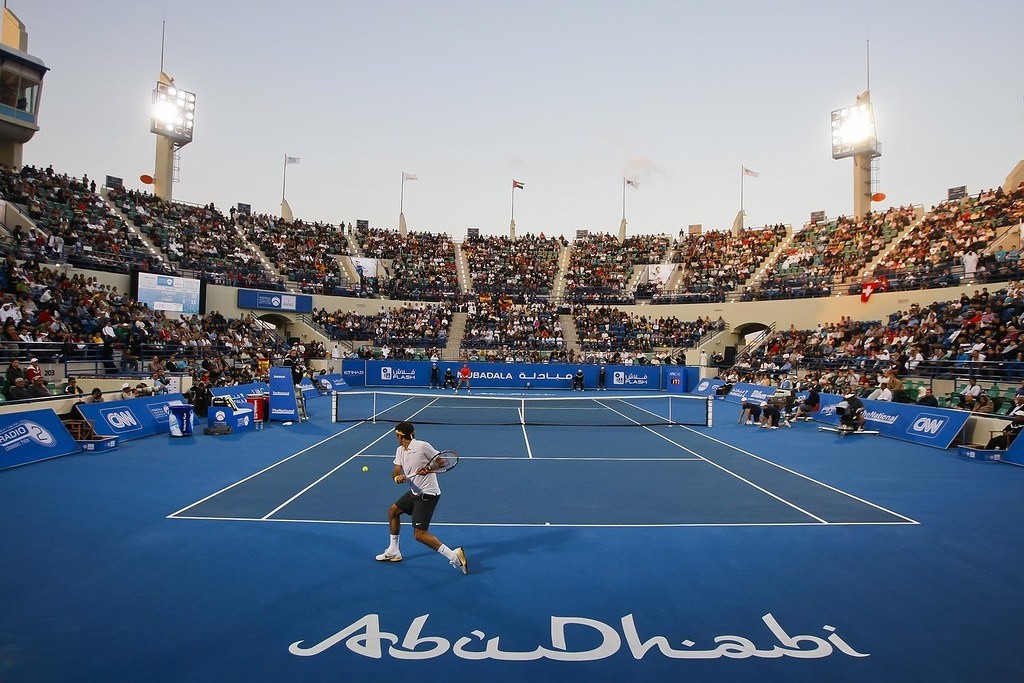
[830,101,882,224]
[151,81,197,204]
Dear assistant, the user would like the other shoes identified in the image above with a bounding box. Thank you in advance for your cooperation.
[784,420,791,428]
[573,389,575,392]
[762,423,768,427]
[790,418,797,423]
[597,388,599,390]
[604,388,606,391]
[803,418,808,421]
[745,420,752,424]
[581,389,584,392]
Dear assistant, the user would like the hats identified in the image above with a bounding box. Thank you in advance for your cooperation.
[760,401,768,406]
[31,358,39,362]
[1013,410,1024,417]
[741,397,747,401]
[120,383,130,390]
[601,367,604,369]
[578,370,582,372]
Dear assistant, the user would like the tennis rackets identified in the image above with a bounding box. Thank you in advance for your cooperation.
[406,449,459,480]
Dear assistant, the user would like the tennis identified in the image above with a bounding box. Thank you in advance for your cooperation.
[362,466,368,472]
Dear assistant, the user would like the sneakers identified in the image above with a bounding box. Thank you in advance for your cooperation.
[376,550,402,562]
[449,547,468,575]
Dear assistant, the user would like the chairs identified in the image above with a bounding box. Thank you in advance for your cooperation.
[787,320,883,333]
[501,246,558,267]
[599,325,648,350]
[62,419,96,440]
[116,199,189,262]
[37,187,120,238]
[803,402,819,421]
[774,221,898,283]
[907,197,992,267]
[901,379,1017,449]
[46,383,67,396]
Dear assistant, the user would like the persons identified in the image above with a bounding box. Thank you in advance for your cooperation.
[372,422,469,576]
[0,146,1024,454]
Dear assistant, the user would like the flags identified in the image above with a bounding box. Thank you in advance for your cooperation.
[284,155,301,165]
[742,167,759,179]
[498,299,511,307]
[480,294,492,304]
[402,172,420,181]
[511,179,526,190]
[860,283,876,303]
[623,176,642,190]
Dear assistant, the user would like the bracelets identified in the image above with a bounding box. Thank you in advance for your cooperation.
[394,476,398,485]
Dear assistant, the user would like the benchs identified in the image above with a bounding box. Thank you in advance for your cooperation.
[208,395,255,433]
